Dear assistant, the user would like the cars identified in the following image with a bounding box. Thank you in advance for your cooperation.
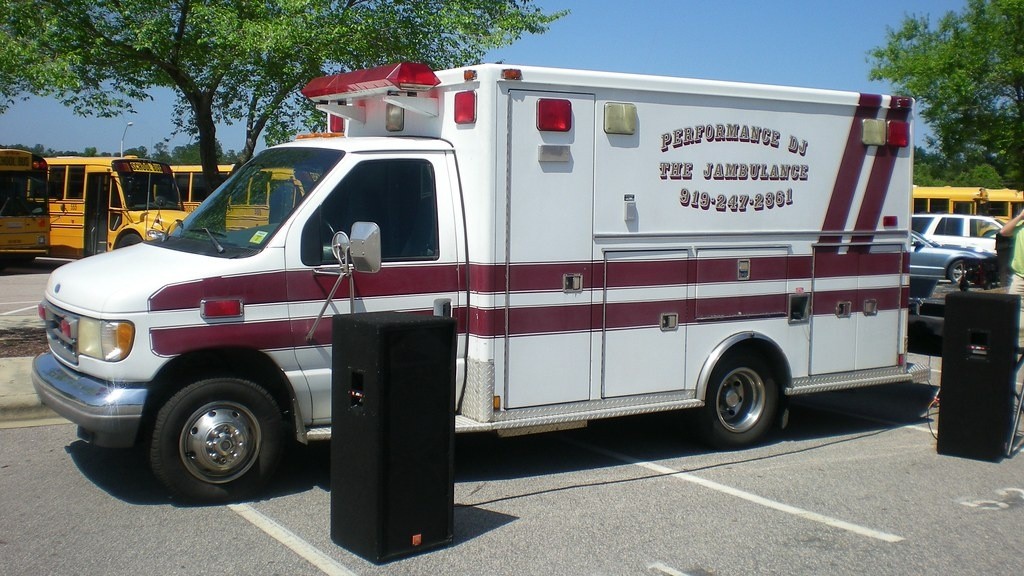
[910,213,1008,285]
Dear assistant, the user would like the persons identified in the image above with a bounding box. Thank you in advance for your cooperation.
[999,209,1024,331]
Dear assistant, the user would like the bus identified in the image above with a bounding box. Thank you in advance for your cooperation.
[150,163,321,236]
[0,148,51,264]
[41,154,191,261]
[911,184,1024,239]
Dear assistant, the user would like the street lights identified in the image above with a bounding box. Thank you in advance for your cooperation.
[121,121,133,156]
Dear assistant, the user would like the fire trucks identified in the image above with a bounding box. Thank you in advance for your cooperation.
[29,59,936,505]
